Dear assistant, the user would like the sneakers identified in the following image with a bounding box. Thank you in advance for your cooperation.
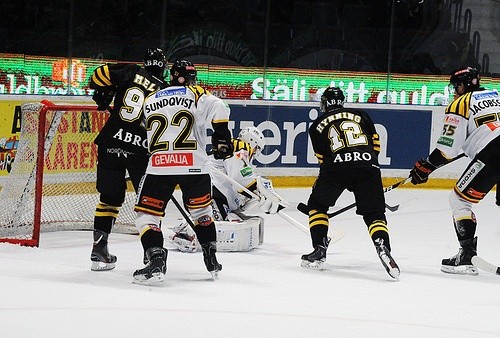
[132,246,168,283]
[202,243,222,281]
[441,237,478,275]
[374,238,400,279]
[90,228,117,271]
[301,237,331,269]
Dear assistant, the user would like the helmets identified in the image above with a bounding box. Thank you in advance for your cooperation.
[450,65,480,91]
[320,86,345,112]
[144,48,165,72]
[170,60,197,83]
[239,127,265,150]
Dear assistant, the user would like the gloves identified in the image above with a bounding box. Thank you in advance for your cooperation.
[410,158,434,185]
[92,87,115,111]
[212,129,233,160]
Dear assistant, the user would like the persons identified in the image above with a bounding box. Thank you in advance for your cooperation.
[301,87,401,281]
[88,49,170,271]
[170,126,285,252]
[409,68,500,274]
[132,61,233,285]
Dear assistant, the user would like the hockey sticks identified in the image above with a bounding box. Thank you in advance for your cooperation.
[385,196,418,212]
[106,104,197,236]
[296,152,468,220]
[210,165,355,246]
[465,249,500,276]
